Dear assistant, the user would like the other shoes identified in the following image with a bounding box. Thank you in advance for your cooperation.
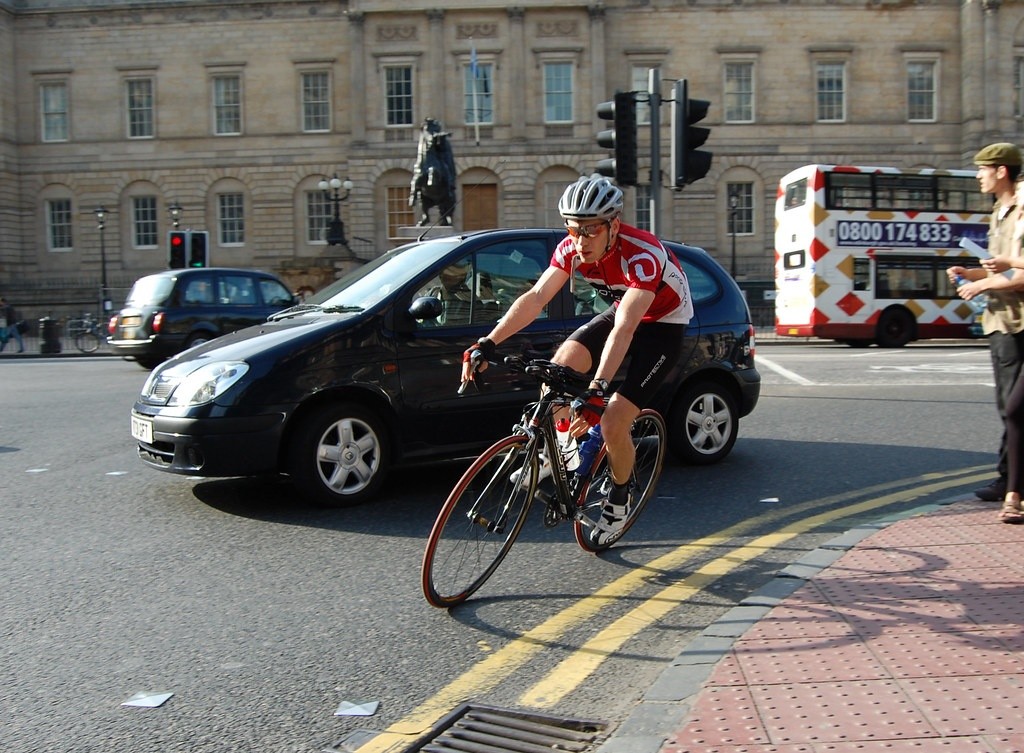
[973,477,1006,501]
[998,500,1024,521]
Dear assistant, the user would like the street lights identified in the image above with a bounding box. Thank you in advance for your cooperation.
[726,188,740,280]
[168,201,183,231]
[92,205,111,321]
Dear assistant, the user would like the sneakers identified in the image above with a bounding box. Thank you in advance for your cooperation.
[590,490,633,545]
[509,433,580,487]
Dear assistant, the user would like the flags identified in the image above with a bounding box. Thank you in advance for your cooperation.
[469,36,480,80]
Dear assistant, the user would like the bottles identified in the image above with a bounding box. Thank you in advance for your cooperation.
[555,417,581,471]
[954,276,991,308]
[574,424,602,475]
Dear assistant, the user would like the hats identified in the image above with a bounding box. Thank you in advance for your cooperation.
[973,142,1022,166]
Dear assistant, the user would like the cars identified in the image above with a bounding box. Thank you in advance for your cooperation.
[106,268,292,371]
[129,225,763,512]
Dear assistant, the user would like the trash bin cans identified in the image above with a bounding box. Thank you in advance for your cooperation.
[38,317,64,354]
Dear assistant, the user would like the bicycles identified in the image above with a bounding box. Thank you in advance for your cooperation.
[420,349,668,605]
[74,322,114,354]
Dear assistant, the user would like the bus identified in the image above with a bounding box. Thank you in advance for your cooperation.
[773,163,996,349]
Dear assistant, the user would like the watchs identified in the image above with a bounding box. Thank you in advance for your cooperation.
[590,378,610,393]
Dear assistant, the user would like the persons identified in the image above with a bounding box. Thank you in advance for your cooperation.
[427,260,548,326]
[461,174,694,544]
[947,142,1024,521]
[920,282,929,290]
[0,298,25,354]
[191,262,207,301]
[878,271,889,290]
[409,117,456,207]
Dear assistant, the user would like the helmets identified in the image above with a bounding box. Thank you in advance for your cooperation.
[558,173,624,222]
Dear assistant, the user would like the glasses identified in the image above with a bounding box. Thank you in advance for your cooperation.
[564,217,616,238]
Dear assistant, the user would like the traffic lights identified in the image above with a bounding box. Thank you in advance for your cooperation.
[187,231,209,268]
[596,91,638,189]
[673,78,714,191]
[166,228,186,268]
